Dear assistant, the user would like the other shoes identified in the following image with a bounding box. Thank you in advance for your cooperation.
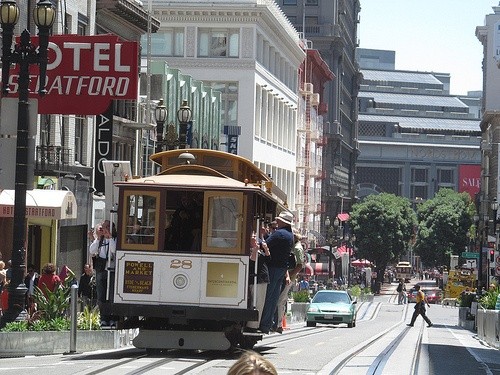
[427,323,433,327]
[244,327,256,333]
[270,327,283,334]
[406,323,414,327]
[99,320,118,327]
[255,328,265,333]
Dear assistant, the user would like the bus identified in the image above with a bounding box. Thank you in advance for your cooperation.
[395,262,412,283]
[99,148,296,355]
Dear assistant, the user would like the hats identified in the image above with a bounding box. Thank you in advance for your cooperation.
[275,211,295,226]
[413,284,420,289]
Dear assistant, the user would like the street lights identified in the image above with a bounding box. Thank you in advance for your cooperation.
[0,0,58,325]
[468,261,475,272]
[325,217,339,287]
[154,98,191,153]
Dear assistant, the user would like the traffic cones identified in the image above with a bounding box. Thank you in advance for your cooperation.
[282,316,290,331]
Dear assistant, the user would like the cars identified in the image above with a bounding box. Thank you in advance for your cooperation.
[422,268,443,280]
[407,282,442,304]
[305,289,357,328]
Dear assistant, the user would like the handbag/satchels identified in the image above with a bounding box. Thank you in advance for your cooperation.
[287,252,297,270]
[94,254,107,272]
[397,285,402,292]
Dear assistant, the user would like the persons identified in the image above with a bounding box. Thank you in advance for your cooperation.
[419,268,436,280]
[0,250,62,330]
[128,216,154,244]
[89,220,117,325]
[292,277,309,292]
[275,227,304,334]
[406,284,433,327]
[165,207,203,251]
[250,211,295,335]
[398,278,408,304]
[78,264,97,316]
[460,284,500,310]
[370,276,376,292]
[312,272,366,291]
[388,268,396,284]
[226,348,279,375]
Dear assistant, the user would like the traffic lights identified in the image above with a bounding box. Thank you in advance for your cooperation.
[490,250,495,263]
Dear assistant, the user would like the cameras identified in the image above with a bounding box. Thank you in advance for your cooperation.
[100,227,104,231]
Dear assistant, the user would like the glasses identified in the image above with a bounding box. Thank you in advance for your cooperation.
[28,270,33,272]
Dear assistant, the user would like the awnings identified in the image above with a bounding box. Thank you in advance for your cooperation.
[338,244,354,253]
[337,212,351,221]
